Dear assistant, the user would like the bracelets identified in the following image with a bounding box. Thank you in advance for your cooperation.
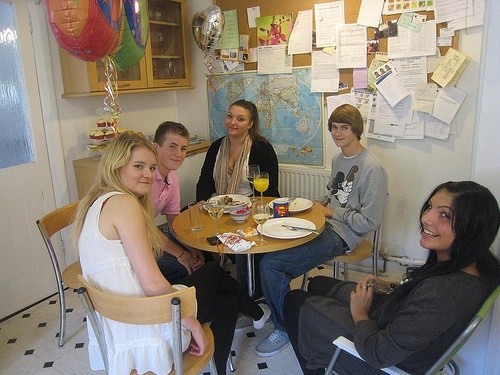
[178,250,186,259]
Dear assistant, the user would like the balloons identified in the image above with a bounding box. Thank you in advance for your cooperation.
[192,5,225,56]
[109,0,150,72]
[43,0,122,62]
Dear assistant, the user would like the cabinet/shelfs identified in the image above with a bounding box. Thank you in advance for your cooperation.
[58,0,196,99]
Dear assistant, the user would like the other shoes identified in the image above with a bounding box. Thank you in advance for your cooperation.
[253,302,272,329]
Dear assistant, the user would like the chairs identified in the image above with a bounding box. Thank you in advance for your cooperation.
[324,286,500,375]
[76,273,214,375]
[36,199,88,347]
[300,214,384,291]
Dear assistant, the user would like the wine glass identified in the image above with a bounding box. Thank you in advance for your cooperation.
[253,172,269,200]
[207,197,224,237]
[252,201,270,246]
[246,165,260,203]
[156,31,168,56]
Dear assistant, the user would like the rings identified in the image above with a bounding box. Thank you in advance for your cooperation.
[362,285,369,288]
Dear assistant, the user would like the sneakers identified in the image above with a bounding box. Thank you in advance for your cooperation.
[234,315,254,333]
[255,330,291,356]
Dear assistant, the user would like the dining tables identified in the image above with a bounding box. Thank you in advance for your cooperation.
[171,197,326,331]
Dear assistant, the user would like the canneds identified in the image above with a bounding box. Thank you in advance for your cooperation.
[273,201,289,218]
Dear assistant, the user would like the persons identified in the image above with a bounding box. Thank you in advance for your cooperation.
[236,102,390,357]
[195,97,280,293]
[282,180,500,375]
[68,131,272,375]
[148,121,217,284]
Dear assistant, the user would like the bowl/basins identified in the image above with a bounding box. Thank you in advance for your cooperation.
[229,208,251,224]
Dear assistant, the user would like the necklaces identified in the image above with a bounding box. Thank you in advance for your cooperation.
[376,276,410,294]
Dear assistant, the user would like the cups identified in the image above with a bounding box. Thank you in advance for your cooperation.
[273,200,290,218]
[165,61,177,79]
[188,202,202,232]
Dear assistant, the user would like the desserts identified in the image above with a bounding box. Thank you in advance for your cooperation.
[89,117,125,150]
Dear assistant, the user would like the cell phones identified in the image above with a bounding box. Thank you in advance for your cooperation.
[206,237,222,245]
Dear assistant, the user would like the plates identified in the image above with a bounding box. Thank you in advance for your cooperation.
[268,198,313,213]
[257,217,316,239]
[203,201,252,213]
[207,195,250,210]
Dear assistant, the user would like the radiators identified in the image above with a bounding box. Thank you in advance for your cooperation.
[278,168,332,204]
